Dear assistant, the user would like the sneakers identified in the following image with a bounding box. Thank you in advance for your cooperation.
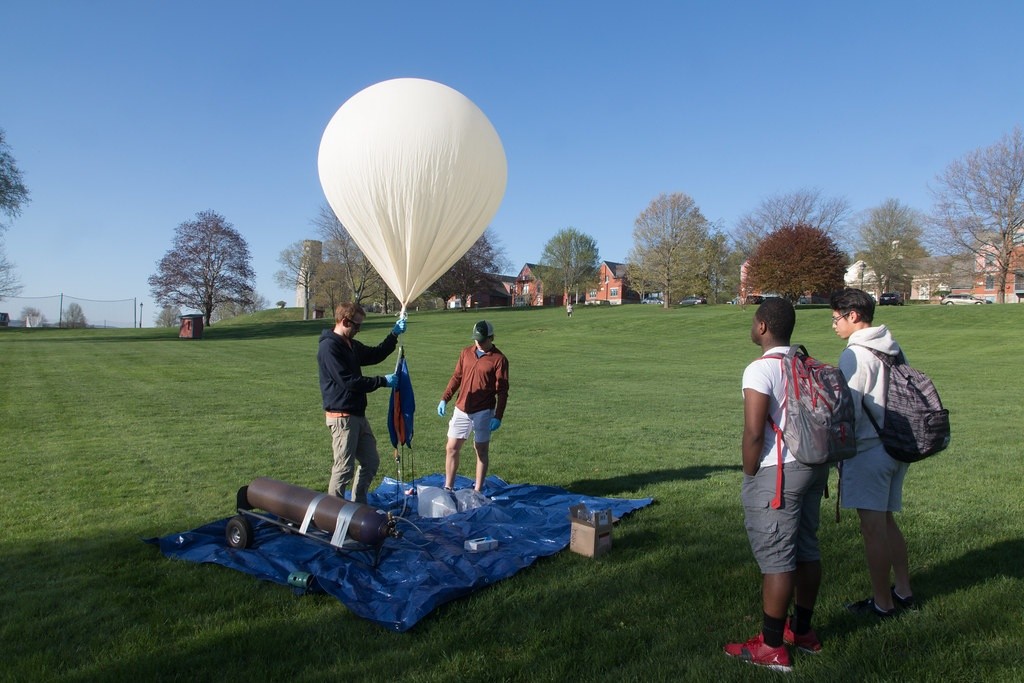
[890,585,918,608]
[782,616,822,653]
[847,597,898,620]
[722,632,794,672]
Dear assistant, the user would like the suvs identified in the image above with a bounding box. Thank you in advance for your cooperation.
[940,293,993,306]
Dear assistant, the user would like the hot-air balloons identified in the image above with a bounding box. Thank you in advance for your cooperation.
[316,77,509,450]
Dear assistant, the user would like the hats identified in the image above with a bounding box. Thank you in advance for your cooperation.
[471,320,494,341]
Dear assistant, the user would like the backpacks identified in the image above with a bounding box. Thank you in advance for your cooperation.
[756,345,857,465]
[844,343,950,463]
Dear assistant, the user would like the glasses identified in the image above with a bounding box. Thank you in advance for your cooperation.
[831,312,860,325]
[341,317,361,329]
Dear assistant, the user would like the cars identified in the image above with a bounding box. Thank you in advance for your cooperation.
[879,292,904,307]
[678,296,706,305]
[641,297,664,305]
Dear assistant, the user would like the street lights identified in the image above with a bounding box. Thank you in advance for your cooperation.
[139,302,143,328]
[859,262,866,291]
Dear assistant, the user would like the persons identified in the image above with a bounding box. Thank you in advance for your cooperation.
[318,302,406,503]
[567,303,573,317]
[830,287,918,620]
[722,298,829,670]
[437,319,509,492]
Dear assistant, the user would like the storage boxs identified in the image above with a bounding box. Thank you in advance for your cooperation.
[566,505,619,557]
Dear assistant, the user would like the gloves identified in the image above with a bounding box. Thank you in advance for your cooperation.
[489,418,501,431]
[437,401,447,416]
[384,373,399,388]
[393,319,407,336]
